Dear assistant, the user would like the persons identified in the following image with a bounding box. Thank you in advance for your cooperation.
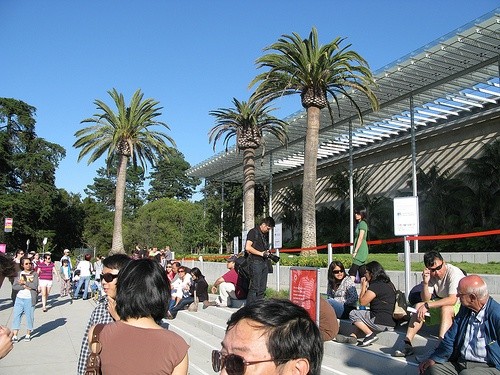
[59,249,104,300]
[348,206,368,283]
[418,275,500,375]
[0,252,18,360]
[78,254,132,375]
[211,255,250,306]
[212,299,324,375]
[392,250,466,363]
[325,261,357,320]
[89,259,191,375]
[133,244,209,320]
[11,250,24,300]
[37,254,59,313]
[24,251,40,269]
[245,217,275,302]
[320,299,339,341]
[336,261,397,347]
[11,257,39,343]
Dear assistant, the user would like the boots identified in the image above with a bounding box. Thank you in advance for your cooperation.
[203,300,217,309]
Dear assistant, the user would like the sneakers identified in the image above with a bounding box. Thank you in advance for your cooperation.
[25,334,30,341]
[394,340,414,356]
[415,346,437,363]
[12,336,18,342]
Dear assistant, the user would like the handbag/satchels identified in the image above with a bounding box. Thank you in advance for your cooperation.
[393,293,407,319]
[234,255,250,279]
[188,301,205,312]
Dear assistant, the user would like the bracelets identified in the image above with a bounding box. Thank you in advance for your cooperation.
[353,252,357,253]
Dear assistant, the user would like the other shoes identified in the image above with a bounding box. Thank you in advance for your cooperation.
[168,309,176,319]
[335,334,357,344]
[358,333,378,346]
[43,307,47,312]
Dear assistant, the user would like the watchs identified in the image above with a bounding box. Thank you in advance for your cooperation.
[424,302,429,309]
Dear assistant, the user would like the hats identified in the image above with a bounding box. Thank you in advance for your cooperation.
[224,256,237,262]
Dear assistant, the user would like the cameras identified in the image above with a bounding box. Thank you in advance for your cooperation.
[268,252,280,263]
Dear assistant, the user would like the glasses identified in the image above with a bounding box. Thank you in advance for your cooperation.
[47,256,51,260]
[191,274,195,277]
[354,212,360,215]
[211,350,300,375]
[178,270,184,273]
[456,291,477,298]
[24,263,31,265]
[99,273,118,282]
[429,259,443,272]
[332,269,344,274]
